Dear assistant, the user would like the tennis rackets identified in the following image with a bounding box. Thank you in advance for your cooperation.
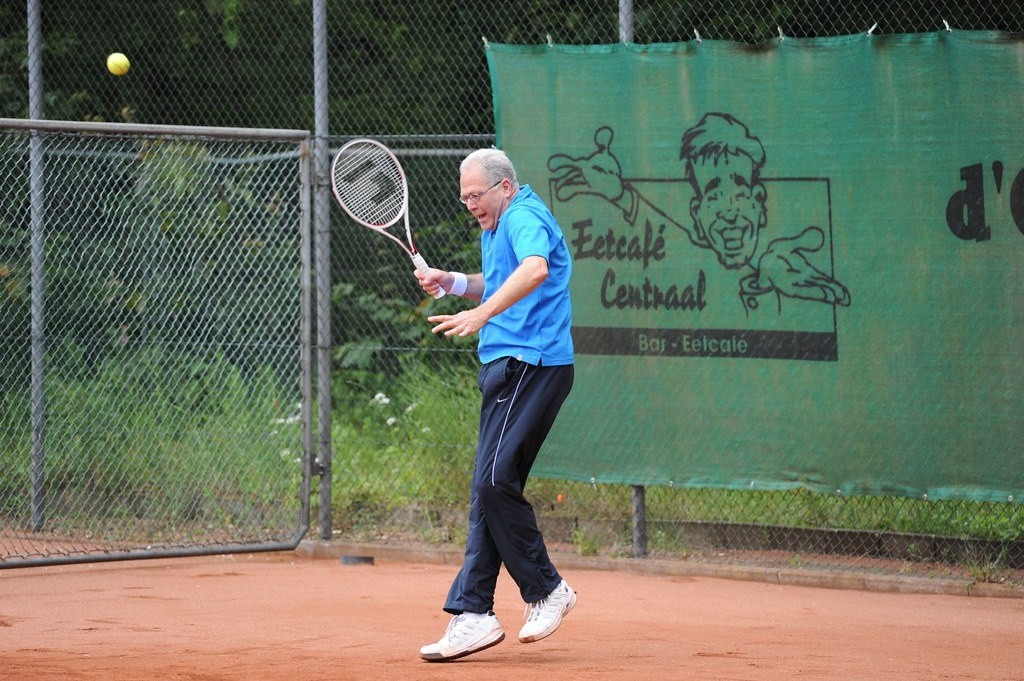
[328,136,448,301]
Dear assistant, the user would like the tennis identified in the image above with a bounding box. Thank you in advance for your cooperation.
[106,52,131,76]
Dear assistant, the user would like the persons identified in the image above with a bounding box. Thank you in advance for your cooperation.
[410,146,578,664]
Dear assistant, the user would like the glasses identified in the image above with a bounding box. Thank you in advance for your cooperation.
[459,178,505,204]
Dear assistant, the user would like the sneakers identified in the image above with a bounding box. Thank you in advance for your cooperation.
[420,611,504,661]
[518,579,578,644]
